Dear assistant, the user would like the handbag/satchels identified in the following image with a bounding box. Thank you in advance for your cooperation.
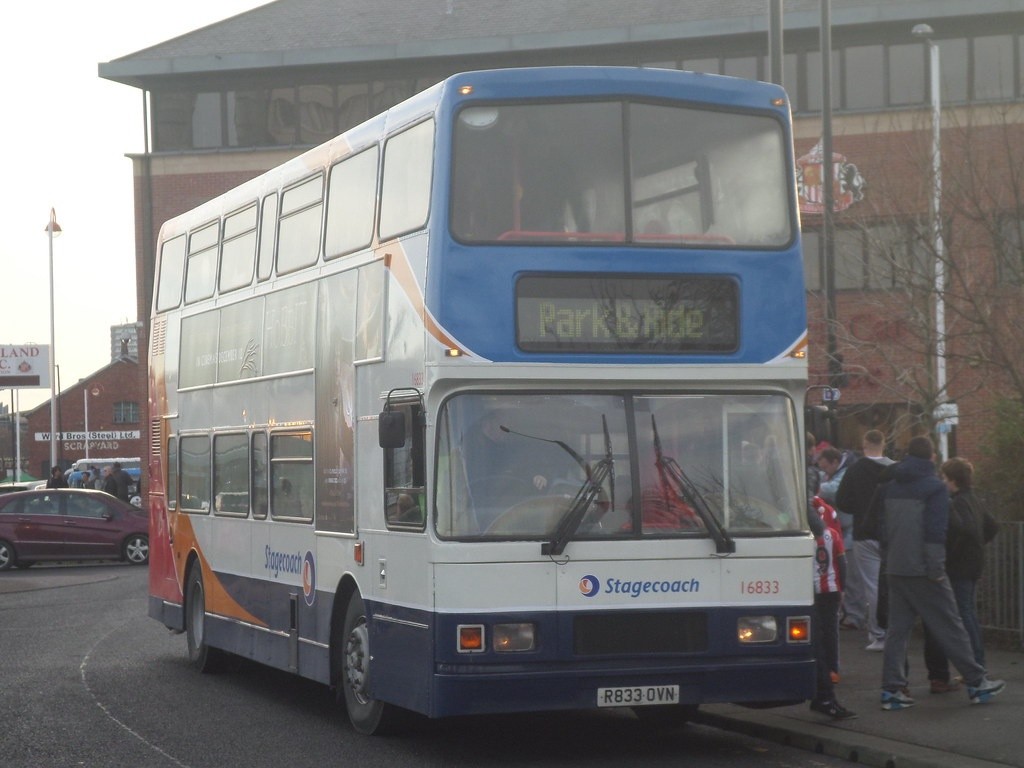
[981,503,999,545]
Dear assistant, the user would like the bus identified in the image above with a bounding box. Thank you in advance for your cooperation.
[66,457,141,495]
[145,60,821,739]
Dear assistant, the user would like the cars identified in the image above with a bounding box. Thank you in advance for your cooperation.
[1,482,35,494]
[0,488,150,571]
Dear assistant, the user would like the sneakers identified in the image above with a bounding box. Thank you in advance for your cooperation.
[881,690,916,710]
[966,673,1006,703]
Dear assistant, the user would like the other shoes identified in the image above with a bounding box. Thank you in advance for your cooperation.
[953,675,968,684]
[901,687,910,696]
[830,671,841,683]
[865,639,885,651]
[810,696,859,720]
[839,617,857,630]
[931,679,960,693]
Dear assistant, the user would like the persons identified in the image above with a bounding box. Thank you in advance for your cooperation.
[46,462,141,503]
[737,430,1007,723]
[514,438,590,508]
[637,441,703,527]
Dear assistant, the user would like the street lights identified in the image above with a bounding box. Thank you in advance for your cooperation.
[83,387,101,459]
[908,20,961,465]
[44,207,64,487]
